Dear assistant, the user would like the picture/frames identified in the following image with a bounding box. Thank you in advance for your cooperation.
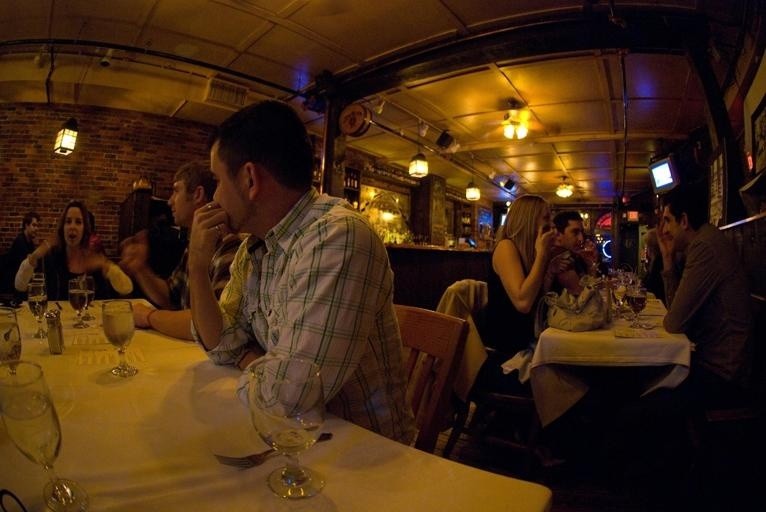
[751,93,766,177]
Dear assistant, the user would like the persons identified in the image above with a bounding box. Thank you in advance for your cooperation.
[13,199,135,302]
[470,189,761,478]
[186,97,420,449]
[87,211,108,258]
[116,160,252,344]
[0,211,38,307]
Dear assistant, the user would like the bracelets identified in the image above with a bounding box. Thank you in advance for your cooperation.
[38,237,53,253]
[24,252,36,266]
[147,307,159,329]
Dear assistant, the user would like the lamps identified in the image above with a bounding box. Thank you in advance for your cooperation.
[53,81,82,156]
[409,114,429,178]
[500,98,530,140]
[555,176,574,199]
[100,48,115,68]
[465,152,481,201]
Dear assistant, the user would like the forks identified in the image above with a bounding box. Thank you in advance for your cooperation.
[215,432,335,469]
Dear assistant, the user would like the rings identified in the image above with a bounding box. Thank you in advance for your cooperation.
[215,225,222,237]
[206,202,214,211]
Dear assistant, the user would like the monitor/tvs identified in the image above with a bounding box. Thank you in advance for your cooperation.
[648,154,694,194]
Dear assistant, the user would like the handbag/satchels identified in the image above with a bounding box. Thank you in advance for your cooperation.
[547,285,606,331]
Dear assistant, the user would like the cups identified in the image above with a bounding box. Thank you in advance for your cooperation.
[376,225,430,248]
[0,307,21,360]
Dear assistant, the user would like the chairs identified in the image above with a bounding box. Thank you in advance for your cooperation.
[436,278,545,481]
[697,293,766,435]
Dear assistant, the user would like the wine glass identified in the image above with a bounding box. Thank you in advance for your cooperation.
[609,268,648,327]
[0,361,90,512]
[79,276,96,320]
[28,283,48,338]
[249,358,326,499]
[101,300,138,376]
[69,279,89,328]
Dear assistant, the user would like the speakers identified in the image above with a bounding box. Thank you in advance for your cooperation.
[504,179,515,189]
[436,132,454,150]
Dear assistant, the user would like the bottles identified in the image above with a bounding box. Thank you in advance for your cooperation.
[345,169,360,189]
[460,209,473,226]
[50,309,64,347]
[44,310,61,355]
